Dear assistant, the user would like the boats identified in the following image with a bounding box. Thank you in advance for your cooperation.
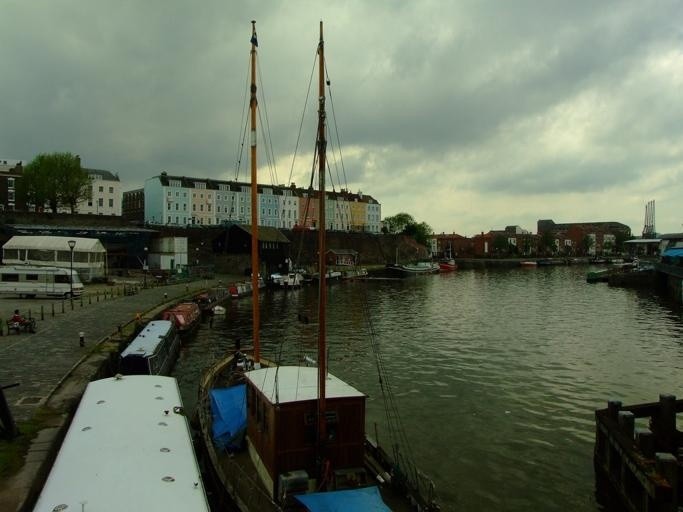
[117,316,182,376]
[269,257,458,287]
[27,373,216,512]
[195,280,231,314]
[520,255,627,266]
[164,300,200,336]
[229,271,266,298]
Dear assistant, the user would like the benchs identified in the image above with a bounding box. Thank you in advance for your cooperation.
[6,314,36,335]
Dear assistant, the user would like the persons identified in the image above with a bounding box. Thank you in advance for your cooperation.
[10,309,36,333]
[80,330,85,347]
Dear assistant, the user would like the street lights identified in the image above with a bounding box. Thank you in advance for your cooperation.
[68,241,76,304]
[143,246,148,289]
[95,198,99,215]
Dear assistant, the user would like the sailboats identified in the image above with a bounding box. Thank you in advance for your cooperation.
[197,18,446,512]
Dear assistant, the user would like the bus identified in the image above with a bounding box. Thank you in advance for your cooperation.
[0,264,84,298]
